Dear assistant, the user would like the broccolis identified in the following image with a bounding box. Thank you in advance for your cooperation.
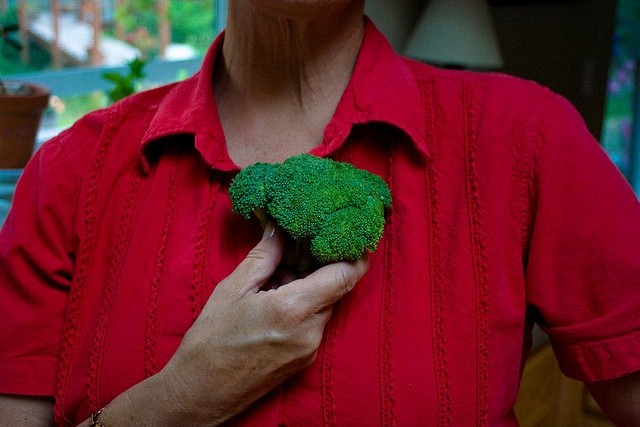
[228,154,392,264]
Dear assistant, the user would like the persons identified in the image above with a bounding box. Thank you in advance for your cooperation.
[0,0,640,427]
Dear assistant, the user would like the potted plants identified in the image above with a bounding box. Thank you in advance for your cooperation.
[1,79,51,166]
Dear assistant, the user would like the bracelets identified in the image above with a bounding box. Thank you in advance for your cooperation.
[89,406,104,427]
